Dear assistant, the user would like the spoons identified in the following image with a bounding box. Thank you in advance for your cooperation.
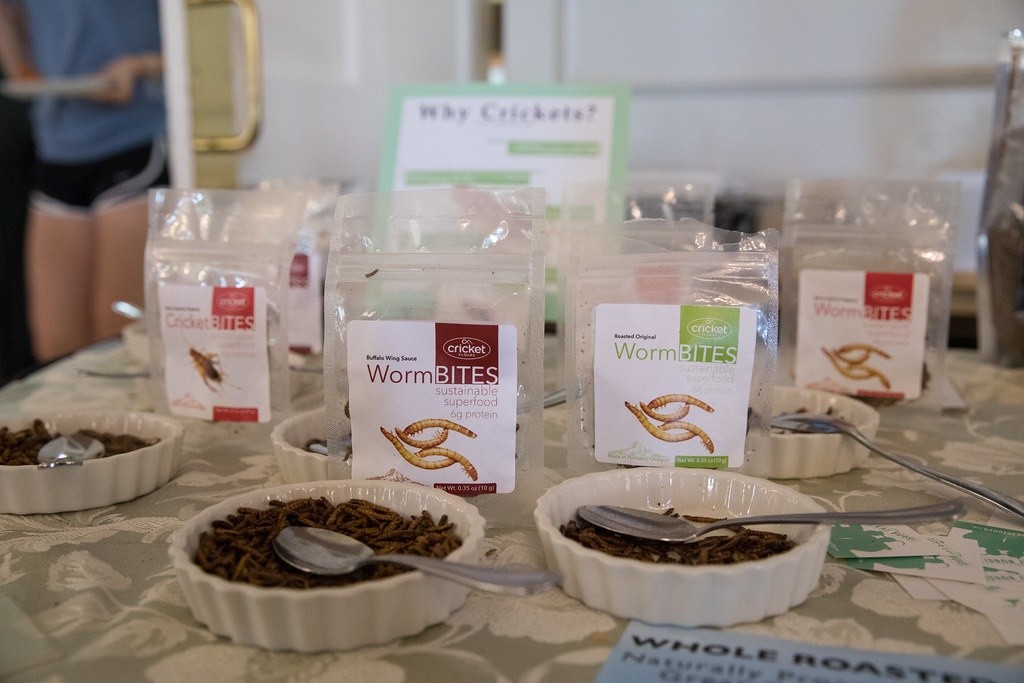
[771,412,1024,520]
[577,501,967,542]
[272,526,562,597]
[38,435,106,468]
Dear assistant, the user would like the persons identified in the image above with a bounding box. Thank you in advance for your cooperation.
[0,0,171,365]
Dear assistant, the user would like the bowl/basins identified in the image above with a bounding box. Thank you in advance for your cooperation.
[167,479,488,653]
[0,408,185,516]
[748,386,881,481]
[269,406,330,482]
[533,466,832,627]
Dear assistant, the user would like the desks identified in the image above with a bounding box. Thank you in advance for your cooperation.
[0,322,1024,683]
